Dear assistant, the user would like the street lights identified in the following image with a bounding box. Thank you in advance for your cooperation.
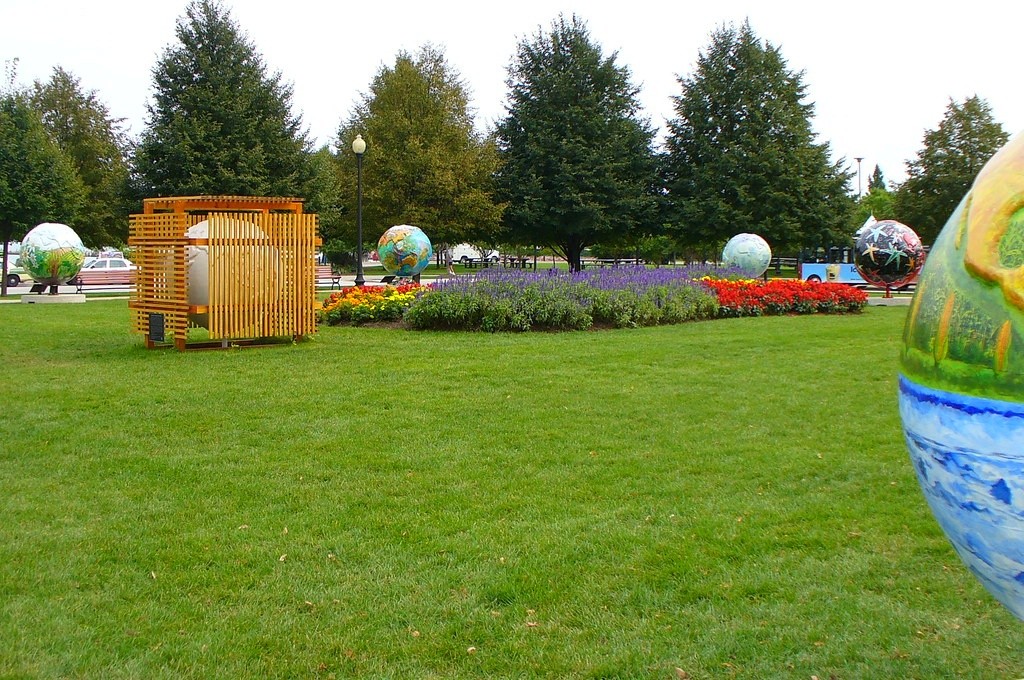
[853,156,865,202]
[352,134,366,287]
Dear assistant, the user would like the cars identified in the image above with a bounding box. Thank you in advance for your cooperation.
[80,258,141,272]
[444,244,501,264]
[0,255,36,288]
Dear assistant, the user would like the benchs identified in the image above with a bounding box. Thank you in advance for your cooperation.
[463,261,533,264]
[287,262,343,290]
[76,269,141,293]
[579,264,640,267]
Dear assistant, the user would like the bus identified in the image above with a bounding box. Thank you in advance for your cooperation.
[799,244,931,291]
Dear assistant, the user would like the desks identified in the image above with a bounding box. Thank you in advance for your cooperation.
[520,257,530,268]
[508,258,518,266]
[580,259,643,270]
[465,258,495,269]
